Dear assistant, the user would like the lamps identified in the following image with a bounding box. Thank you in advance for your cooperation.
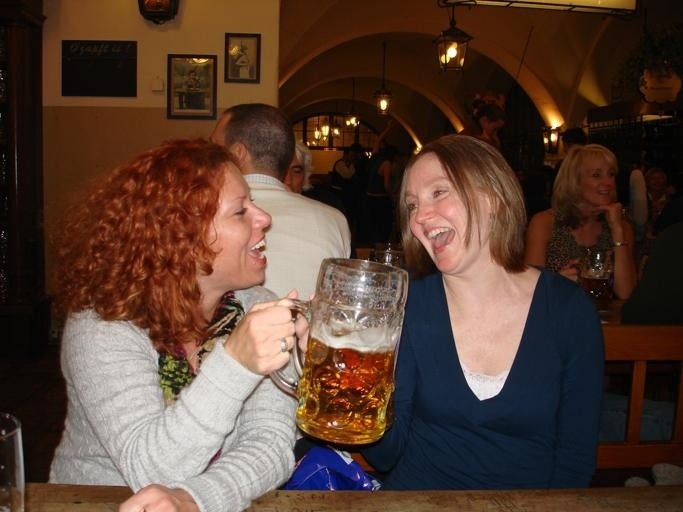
[138,0,179,25]
[314,32,393,142]
[431,0,477,73]
[542,126,564,154]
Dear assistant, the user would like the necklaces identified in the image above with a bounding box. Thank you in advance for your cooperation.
[187,345,200,363]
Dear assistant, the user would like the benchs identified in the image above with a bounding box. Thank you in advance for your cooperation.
[350,325,683,488]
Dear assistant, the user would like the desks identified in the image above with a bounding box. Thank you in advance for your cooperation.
[24,482,682,512]
[602,300,626,325]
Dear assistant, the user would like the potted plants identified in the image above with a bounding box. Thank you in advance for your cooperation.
[622,7,682,104]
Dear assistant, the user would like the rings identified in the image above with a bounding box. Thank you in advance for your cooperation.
[279,338,288,353]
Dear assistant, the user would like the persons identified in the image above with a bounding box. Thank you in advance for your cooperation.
[234,45,249,78]
[45,137,304,512]
[211,104,355,323]
[282,102,682,441]
[182,70,201,109]
[295,132,606,493]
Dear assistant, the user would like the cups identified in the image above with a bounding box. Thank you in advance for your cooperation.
[263,258,408,445]
[568,245,616,314]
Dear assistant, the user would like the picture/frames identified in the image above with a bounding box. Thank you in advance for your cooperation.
[167,54,217,119]
[224,33,261,83]
